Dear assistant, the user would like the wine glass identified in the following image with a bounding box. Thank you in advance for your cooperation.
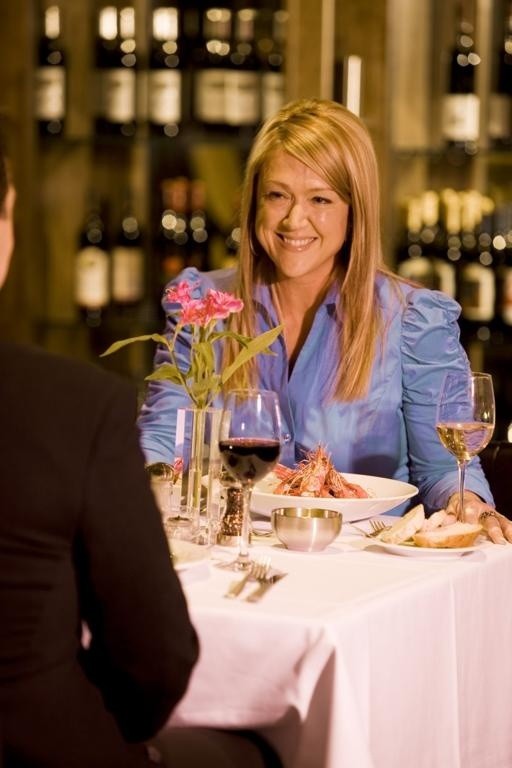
[435,371,497,524]
[219,388,282,573]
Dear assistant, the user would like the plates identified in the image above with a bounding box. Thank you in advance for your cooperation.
[249,471,419,524]
[366,534,487,559]
[168,537,212,572]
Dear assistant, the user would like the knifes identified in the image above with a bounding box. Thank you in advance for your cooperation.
[247,573,288,602]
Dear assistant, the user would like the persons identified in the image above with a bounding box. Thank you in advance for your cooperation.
[135,96,512,546]
[1,124,285,768]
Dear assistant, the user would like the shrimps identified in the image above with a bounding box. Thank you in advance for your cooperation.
[271,437,373,500]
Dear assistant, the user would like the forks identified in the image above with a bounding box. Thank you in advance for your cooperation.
[370,521,388,533]
[226,561,271,599]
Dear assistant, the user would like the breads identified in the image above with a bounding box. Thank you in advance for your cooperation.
[383,504,483,548]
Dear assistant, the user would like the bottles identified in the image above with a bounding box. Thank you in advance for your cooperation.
[32,2,512,343]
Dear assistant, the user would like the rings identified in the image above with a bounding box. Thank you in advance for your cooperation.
[478,510,496,522]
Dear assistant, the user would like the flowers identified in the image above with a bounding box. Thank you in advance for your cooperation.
[99,278,291,516]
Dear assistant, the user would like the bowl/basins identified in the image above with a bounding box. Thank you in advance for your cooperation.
[269,508,343,551]
